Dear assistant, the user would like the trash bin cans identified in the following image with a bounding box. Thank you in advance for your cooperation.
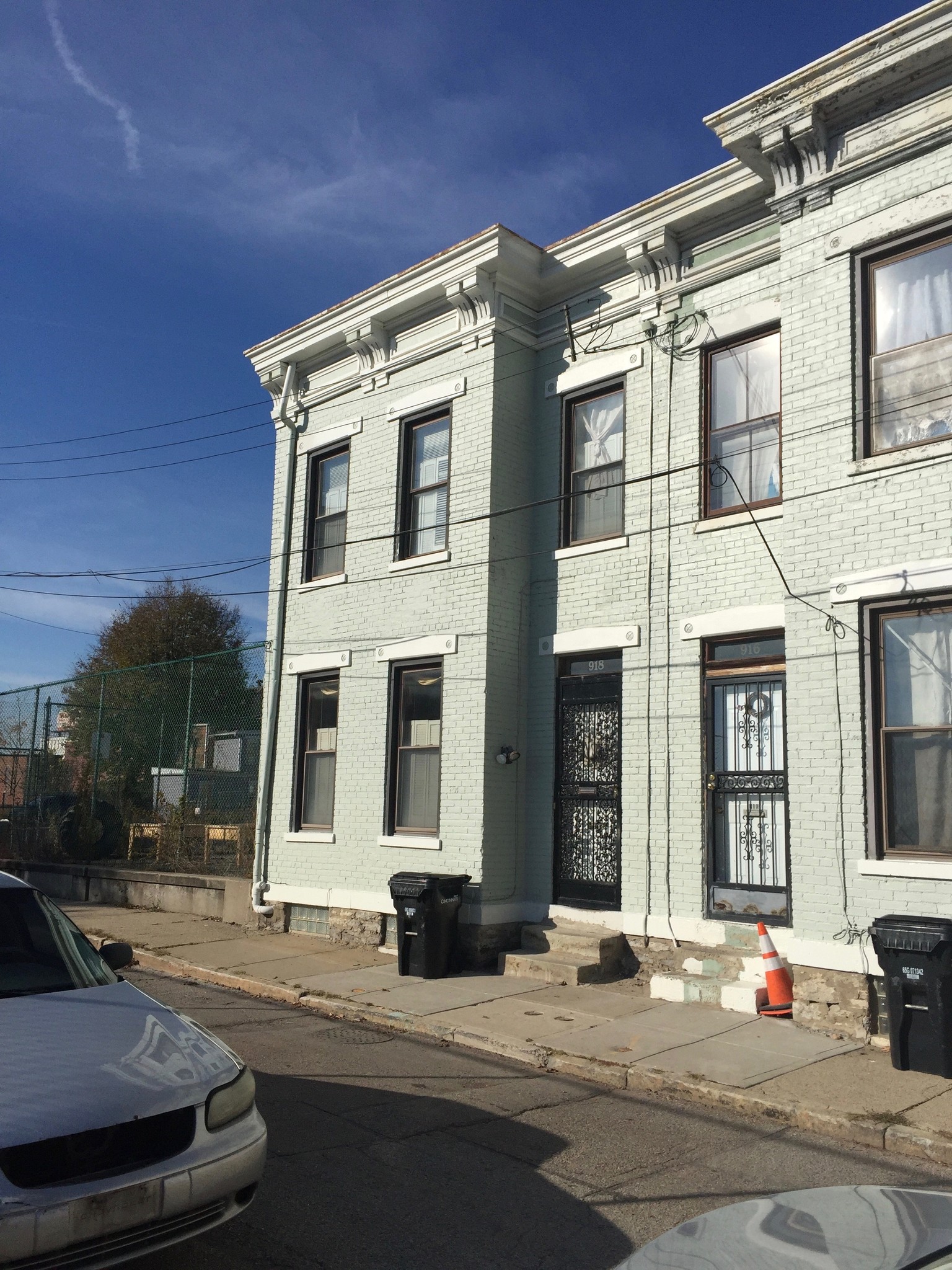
[868,914,952,1078]
[387,871,472,980]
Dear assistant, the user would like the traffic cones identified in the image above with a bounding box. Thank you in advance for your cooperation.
[756,922,793,1016]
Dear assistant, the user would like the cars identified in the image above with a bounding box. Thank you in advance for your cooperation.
[0,872,267,1270]
